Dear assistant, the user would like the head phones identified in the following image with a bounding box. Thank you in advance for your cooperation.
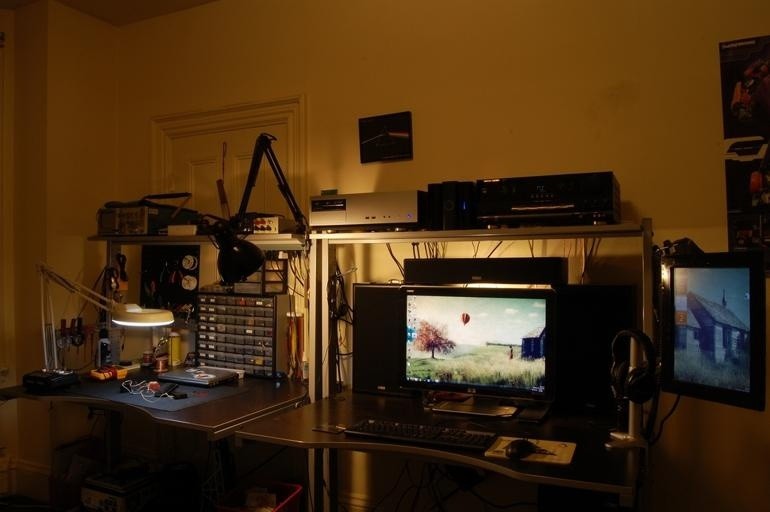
[607,327,657,403]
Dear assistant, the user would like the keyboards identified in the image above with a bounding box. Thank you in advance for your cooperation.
[343,417,499,456]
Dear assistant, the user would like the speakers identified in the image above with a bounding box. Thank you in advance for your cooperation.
[556,281,637,420]
[404,258,570,289]
[350,282,407,398]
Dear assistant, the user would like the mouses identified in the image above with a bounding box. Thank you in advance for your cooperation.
[506,438,536,460]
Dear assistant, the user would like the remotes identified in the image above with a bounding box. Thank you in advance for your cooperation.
[154,383,179,397]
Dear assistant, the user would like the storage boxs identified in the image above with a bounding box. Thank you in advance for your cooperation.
[215,477,305,512]
[81,465,155,511]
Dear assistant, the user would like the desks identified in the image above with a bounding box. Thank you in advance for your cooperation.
[0,363,311,512]
[234,387,655,512]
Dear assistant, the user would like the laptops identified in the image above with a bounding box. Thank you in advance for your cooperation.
[158,365,239,388]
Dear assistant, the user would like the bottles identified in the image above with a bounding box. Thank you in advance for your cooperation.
[96,327,112,368]
[168,326,180,366]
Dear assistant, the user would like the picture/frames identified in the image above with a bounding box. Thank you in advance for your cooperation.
[359,111,414,164]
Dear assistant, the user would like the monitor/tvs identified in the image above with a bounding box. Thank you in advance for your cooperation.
[405,288,555,420]
[664,251,766,411]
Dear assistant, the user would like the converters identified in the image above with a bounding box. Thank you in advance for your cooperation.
[172,391,188,399]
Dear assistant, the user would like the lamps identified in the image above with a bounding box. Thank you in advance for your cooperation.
[22,261,176,390]
[208,133,309,283]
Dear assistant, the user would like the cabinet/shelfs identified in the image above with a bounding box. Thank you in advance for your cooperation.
[194,287,297,386]
